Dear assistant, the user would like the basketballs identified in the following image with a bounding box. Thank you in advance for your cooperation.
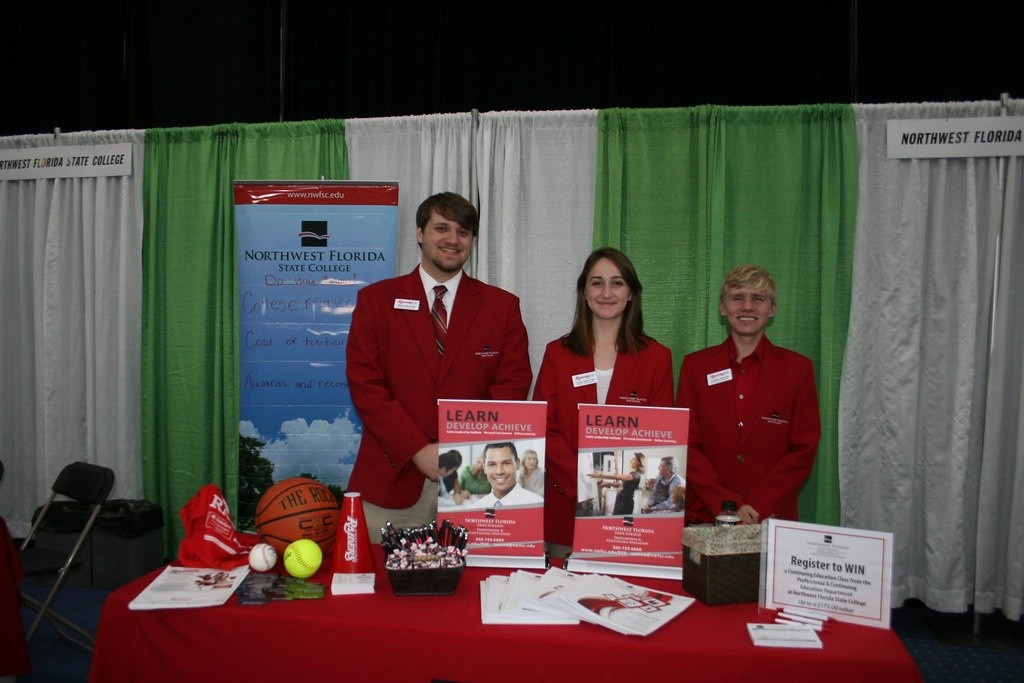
[254,477,340,557]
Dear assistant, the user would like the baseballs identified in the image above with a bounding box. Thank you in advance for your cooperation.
[248,543,278,573]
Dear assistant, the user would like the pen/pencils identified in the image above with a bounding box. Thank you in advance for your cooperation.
[380,517,469,552]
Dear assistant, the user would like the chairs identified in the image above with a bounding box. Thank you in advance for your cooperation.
[15,460,115,657]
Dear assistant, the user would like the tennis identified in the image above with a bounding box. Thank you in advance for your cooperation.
[281,539,324,578]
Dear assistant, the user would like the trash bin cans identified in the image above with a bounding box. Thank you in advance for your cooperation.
[92,498,165,590]
[32,500,91,590]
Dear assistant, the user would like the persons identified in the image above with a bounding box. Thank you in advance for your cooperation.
[346,190,533,544]
[586,452,685,516]
[437,441,544,506]
[532,247,675,558]
[675,264,821,524]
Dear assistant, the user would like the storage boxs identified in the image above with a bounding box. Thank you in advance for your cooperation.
[681,525,761,605]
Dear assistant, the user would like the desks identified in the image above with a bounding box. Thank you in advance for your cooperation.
[87,559,926,683]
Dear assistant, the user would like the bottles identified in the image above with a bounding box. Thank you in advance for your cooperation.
[715,500,743,528]
